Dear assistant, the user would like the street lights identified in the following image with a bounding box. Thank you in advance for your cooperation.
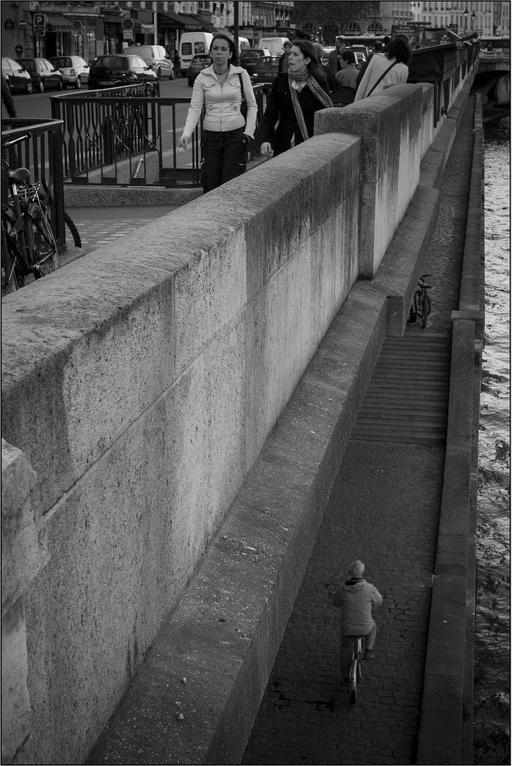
[463,6,469,34]
[471,10,476,32]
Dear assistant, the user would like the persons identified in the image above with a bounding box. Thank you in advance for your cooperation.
[255,35,416,158]
[178,33,259,194]
[1,74,17,118]
[332,559,383,682]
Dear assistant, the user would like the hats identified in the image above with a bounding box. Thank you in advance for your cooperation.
[350,560,365,578]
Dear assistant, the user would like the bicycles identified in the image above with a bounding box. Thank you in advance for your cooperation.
[99,73,160,163]
[414,273,432,329]
[344,634,374,706]
[1,131,60,298]
[37,190,82,247]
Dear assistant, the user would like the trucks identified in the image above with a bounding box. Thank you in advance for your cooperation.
[179,31,250,78]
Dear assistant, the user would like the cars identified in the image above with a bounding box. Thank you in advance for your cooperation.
[84,53,160,102]
[251,55,284,86]
[1,57,34,96]
[186,53,213,86]
[305,24,509,66]
[47,55,91,89]
[13,57,64,93]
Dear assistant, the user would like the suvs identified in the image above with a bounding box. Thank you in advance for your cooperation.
[239,48,273,71]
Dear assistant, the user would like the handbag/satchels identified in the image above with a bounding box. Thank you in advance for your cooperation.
[237,73,259,130]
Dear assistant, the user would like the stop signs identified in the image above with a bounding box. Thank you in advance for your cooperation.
[18,18,28,29]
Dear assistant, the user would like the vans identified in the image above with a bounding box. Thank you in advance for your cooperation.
[255,37,294,67]
[123,45,175,82]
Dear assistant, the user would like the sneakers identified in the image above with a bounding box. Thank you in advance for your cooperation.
[365,651,374,658]
